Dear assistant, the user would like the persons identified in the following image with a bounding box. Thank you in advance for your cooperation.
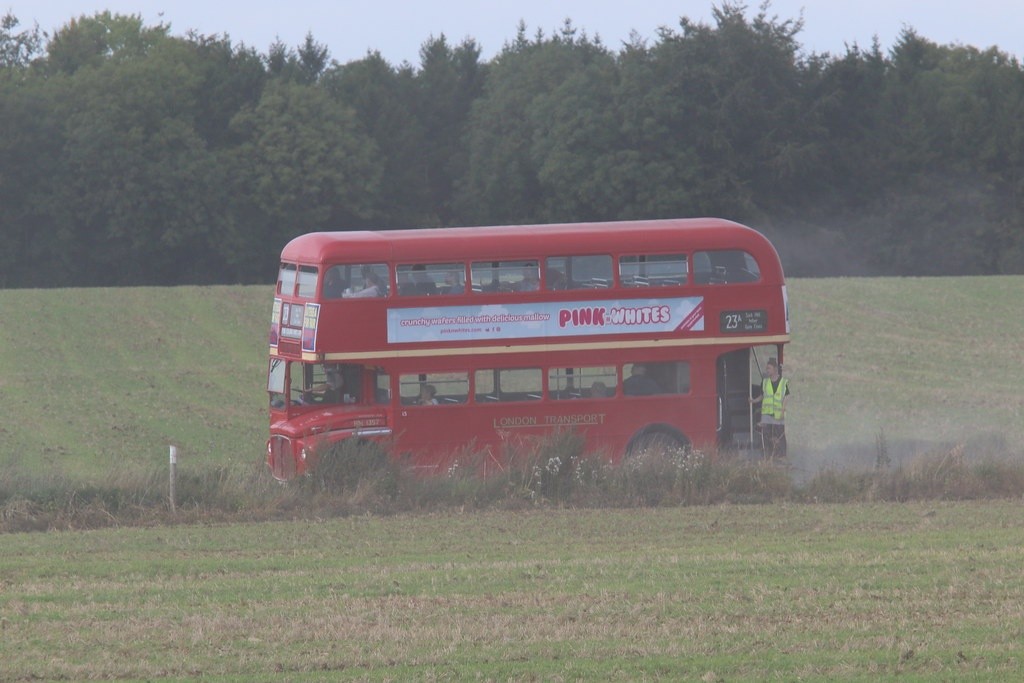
[747,360,789,457]
[512,264,539,291]
[623,364,657,396]
[323,267,386,297]
[422,385,438,405]
[446,271,464,294]
[412,265,435,296]
[305,363,344,402]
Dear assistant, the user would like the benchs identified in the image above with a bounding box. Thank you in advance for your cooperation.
[525,392,543,400]
[573,276,687,290]
[484,396,498,403]
[570,391,582,398]
[442,398,458,404]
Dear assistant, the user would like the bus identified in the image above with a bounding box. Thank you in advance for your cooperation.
[263,217,793,493]
